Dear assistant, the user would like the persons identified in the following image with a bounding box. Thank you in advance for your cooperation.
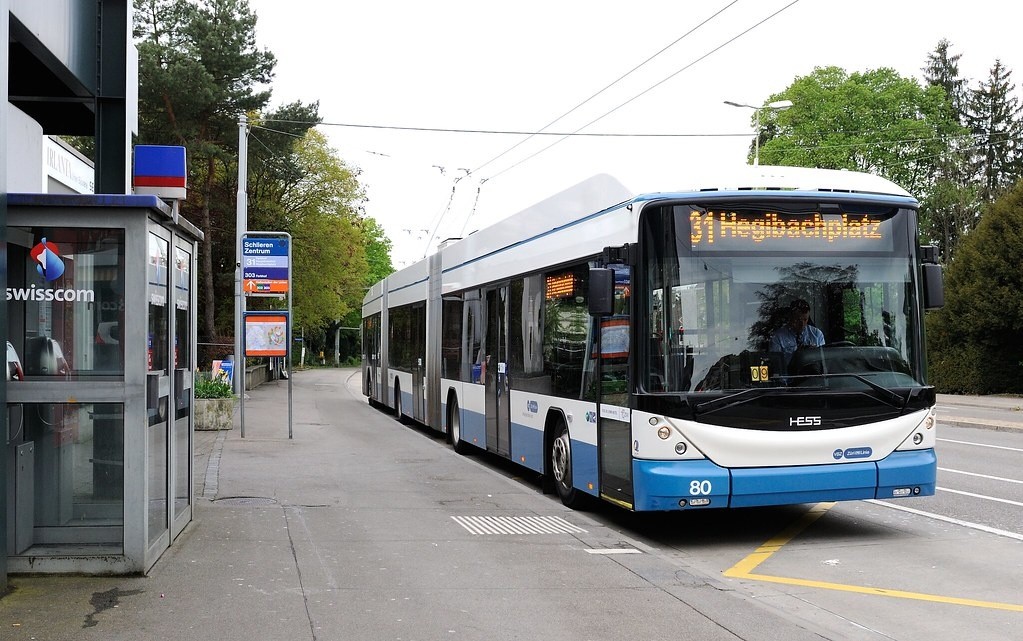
[771,300,825,386]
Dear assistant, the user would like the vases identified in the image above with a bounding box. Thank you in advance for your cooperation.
[194,398,234,430]
[246,365,266,390]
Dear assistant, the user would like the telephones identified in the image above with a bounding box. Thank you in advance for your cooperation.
[6,340,25,405]
[32,337,71,424]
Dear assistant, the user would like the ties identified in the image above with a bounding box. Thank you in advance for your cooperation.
[795,333,804,348]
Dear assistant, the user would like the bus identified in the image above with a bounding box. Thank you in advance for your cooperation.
[358,160,943,513]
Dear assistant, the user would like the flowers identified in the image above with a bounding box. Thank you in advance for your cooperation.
[195,367,233,398]
[246,357,265,367]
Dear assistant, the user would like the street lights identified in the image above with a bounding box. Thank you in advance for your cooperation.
[725,99,799,166]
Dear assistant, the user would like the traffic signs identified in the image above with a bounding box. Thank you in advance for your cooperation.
[243,237,289,293]
